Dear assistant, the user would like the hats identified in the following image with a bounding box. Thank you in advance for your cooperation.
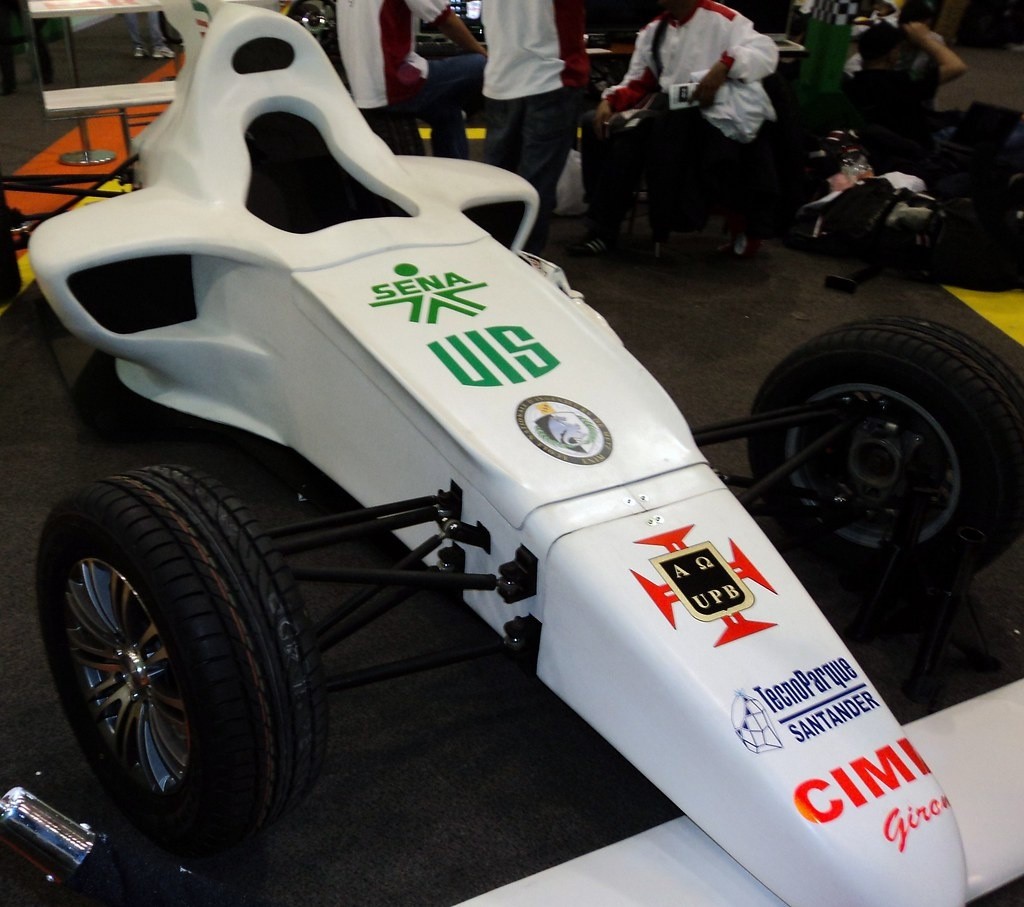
[857,22,907,62]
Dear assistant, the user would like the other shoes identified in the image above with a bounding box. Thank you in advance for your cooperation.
[565,232,626,258]
[134,47,150,57]
[153,46,176,59]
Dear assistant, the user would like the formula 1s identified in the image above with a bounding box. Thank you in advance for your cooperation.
[2,0,1024,906]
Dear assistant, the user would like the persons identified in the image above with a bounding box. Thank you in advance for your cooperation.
[481,0,590,257]
[583,0,780,258]
[891,0,944,114]
[336,0,488,160]
[845,21,968,144]
[125,11,175,58]
